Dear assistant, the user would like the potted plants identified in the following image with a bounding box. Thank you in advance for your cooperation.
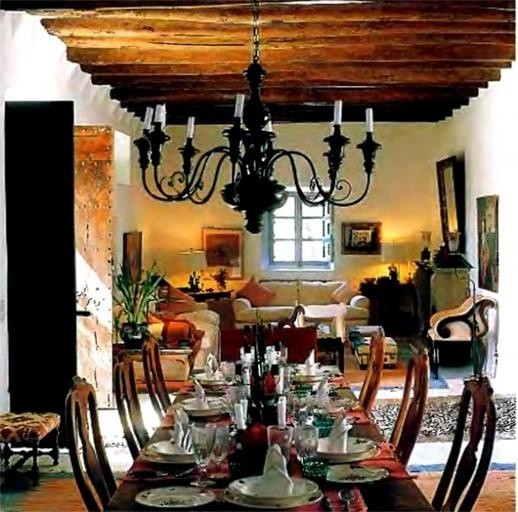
[90,259,172,350]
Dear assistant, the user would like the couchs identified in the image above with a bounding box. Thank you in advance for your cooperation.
[113,300,222,391]
[231,280,370,339]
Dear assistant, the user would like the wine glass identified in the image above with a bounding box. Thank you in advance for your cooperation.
[267,424,294,460]
[207,425,229,479]
[192,424,216,485]
[295,425,318,466]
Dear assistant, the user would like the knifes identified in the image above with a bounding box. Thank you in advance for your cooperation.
[322,495,334,511]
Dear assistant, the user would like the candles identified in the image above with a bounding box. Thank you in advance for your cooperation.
[278,395,287,430]
[233,398,248,430]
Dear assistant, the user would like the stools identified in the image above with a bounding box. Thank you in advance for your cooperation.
[0,412,60,489]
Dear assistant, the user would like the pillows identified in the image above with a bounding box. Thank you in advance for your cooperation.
[330,280,363,305]
[235,274,277,306]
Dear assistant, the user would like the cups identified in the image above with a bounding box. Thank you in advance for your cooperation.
[304,461,329,489]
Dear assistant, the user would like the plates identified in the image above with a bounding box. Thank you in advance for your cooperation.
[221,487,324,509]
[140,449,195,465]
[230,475,319,505]
[134,486,216,508]
[150,439,194,457]
[318,447,381,465]
[316,435,375,459]
[179,362,353,435]
[327,464,387,484]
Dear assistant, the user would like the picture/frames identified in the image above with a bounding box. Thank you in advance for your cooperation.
[122,231,142,283]
[341,222,383,255]
[436,155,466,254]
[201,226,243,280]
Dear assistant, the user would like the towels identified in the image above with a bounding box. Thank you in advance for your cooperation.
[317,374,330,408]
[300,348,321,377]
[328,415,360,454]
[191,378,210,411]
[262,442,295,497]
[205,351,220,380]
[173,408,196,455]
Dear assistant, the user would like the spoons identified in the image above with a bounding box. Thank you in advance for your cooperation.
[339,487,356,512]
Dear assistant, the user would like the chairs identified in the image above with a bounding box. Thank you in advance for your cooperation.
[63,337,172,512]
[422,294,498,380]
[358,333,497,512]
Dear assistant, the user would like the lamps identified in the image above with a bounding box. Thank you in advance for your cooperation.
[133,1,383,236]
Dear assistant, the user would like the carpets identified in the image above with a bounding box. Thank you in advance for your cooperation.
[341,332,449,389]
[0,462,516,512]
[98,393,516,448]
